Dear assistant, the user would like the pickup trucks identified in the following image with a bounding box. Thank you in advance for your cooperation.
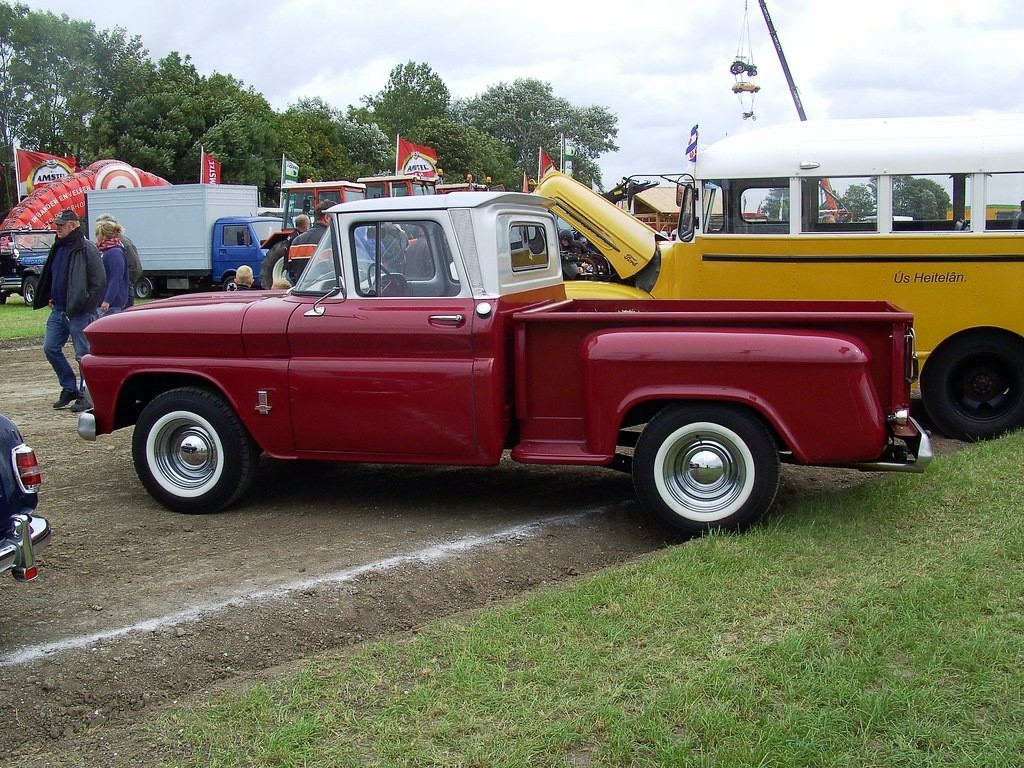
[78,190,933,540]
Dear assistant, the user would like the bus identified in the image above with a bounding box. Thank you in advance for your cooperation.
[517,111,1024,440]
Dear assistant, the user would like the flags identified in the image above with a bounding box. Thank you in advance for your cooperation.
[281,156,299,209]
[397,137,438,178]
[685,125,699,162]
[203,153,221,185]
[541,151,559,179]
[17,148,76,196]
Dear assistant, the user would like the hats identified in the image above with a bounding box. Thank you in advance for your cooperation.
[53,210,79,224]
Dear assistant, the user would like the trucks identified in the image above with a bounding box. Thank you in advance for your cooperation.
[83,178,295,293]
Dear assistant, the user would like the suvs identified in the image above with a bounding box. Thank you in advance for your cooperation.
[0,226,58,308]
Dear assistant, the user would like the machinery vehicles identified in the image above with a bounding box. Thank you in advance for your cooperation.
[259,169,506,291]
[728,0,854,224]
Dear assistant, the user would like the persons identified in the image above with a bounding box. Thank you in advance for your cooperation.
[94,214,142,318]
[822,215,835,223]
[234,265,254,291]
[284,199,588,288]
[33,210,107,413]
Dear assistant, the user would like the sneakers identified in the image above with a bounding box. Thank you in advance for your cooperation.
[70,396,91,412]
[53,389,79,408]
